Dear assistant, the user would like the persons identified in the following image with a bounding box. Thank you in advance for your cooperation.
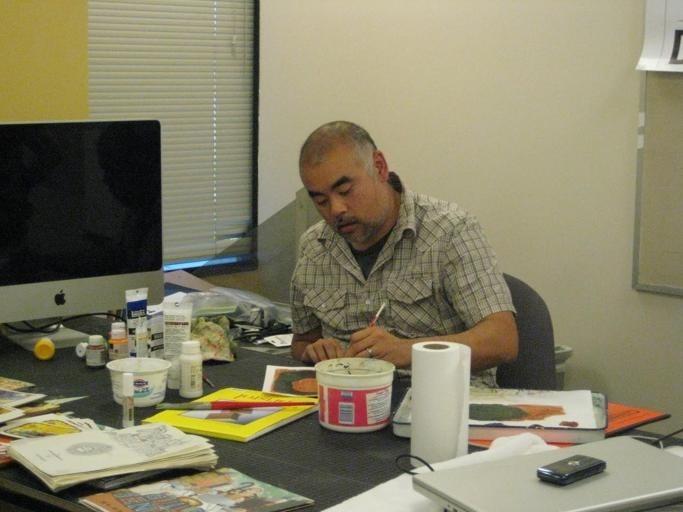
[290,122,520,388]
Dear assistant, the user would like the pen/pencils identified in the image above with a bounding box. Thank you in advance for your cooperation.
[367,302,386,328]
[203,375,215,388]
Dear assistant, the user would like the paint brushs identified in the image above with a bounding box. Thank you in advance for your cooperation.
[155,401,317,410]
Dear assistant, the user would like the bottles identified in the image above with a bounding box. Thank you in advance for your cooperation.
[108,322,126,341]
[108,328,128,361]
[179,340,203,399]
[85,335,106,369]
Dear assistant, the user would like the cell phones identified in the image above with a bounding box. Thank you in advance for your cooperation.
[535,454,607,485]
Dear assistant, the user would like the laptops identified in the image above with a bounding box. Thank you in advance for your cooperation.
[411,434,682,512]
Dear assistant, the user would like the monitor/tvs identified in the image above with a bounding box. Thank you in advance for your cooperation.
[0,118,166,355]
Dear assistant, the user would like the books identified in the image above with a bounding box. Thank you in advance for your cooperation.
[1,372,320,512]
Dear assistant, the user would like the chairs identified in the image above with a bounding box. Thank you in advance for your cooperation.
[493,273,557,389]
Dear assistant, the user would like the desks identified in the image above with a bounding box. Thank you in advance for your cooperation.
[2,309,682,512]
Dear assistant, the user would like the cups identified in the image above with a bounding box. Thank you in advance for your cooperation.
[314,357,396,433]
[104,357,172,407]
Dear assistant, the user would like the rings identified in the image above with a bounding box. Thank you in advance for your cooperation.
[366,347,374,358]
